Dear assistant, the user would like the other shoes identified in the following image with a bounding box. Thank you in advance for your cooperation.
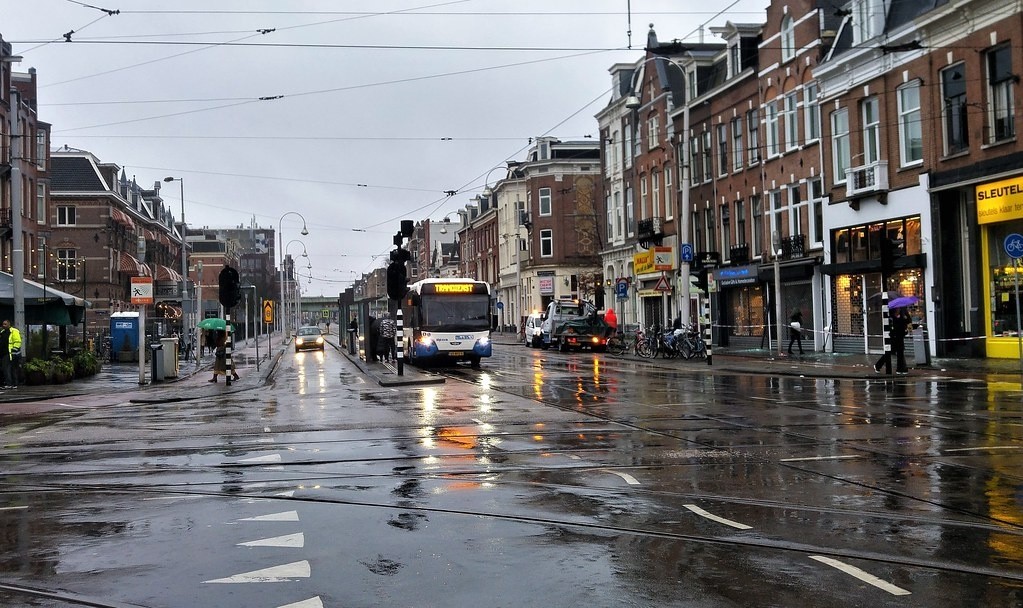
[385,360,390,363]
[392,359,395,363]
[5,384,12,388]
[874,363,880,373]
[385,358,390,361]
[0,386,6,389]
[13,386,18,389]
[379,359,383,363]
[896,370,910,375]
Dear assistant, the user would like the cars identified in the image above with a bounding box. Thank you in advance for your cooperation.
[524,297,617,352]
[292,325,325,353]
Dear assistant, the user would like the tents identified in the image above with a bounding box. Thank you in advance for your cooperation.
[0,271,92,363]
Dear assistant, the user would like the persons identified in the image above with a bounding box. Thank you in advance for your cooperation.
[0,319,22,389]
[369,313,396,362]
[674,311,681,330]
[788,306,805,354]
[208,329,239,383]
[351,318,358,338]
[604,309,617,338]
[200,327,216,358]
[874,306,912,374]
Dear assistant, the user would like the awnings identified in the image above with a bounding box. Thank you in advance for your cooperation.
[119,252,194,282]
[112,207,171,245]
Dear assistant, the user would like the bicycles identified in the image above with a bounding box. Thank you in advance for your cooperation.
[606,324,708,362]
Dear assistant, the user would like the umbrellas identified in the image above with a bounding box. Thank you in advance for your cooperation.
[887,296,920,309]
[196,318,236,332]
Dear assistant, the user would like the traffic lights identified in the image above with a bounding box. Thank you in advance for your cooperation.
[218,267,241,308]
[387,261,410,301]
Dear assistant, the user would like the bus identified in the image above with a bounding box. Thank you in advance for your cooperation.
[385,277,493,371]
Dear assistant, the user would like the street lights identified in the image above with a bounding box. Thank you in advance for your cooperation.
[440,211,469,278]
[163,176,191,358]
[285,239,308,338]
[293,253,312,336]
[278,211,309,345]
[482,166,523,343]
[625,55,691,359]
[407,238,428,279]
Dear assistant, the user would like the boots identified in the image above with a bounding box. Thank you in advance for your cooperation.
[208,373,218,383]
[230,372,239,381]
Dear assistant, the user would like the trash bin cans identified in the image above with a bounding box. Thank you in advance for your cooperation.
[911,323,930,366]
[150,345,165,382]
